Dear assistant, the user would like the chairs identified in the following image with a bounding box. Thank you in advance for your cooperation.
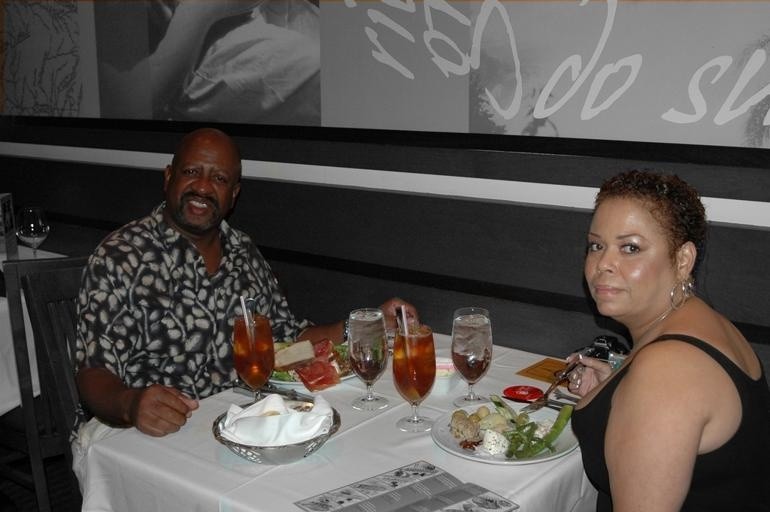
[1,254,91,512]
[18,267,92,512]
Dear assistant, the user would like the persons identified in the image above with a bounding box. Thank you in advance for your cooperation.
[66,127,420,446]
[565,169,770,512]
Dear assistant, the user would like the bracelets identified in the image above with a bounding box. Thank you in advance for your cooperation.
[344,318,349,340]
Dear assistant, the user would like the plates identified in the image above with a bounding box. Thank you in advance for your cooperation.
[267,371,354,385]
[431,402,580,466]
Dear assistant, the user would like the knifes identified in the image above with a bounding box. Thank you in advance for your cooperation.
[232,379,315,404]
[502,394,562,411]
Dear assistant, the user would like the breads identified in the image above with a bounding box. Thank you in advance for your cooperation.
[273,340,316,372]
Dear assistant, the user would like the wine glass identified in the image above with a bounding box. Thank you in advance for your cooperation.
[232,316,275,401]
[348,308,390,411]
[15,206,51,258]
[391,324,438,433]
[451,306,492,409]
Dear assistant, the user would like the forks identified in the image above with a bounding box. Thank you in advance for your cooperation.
[523,346,594,413]
